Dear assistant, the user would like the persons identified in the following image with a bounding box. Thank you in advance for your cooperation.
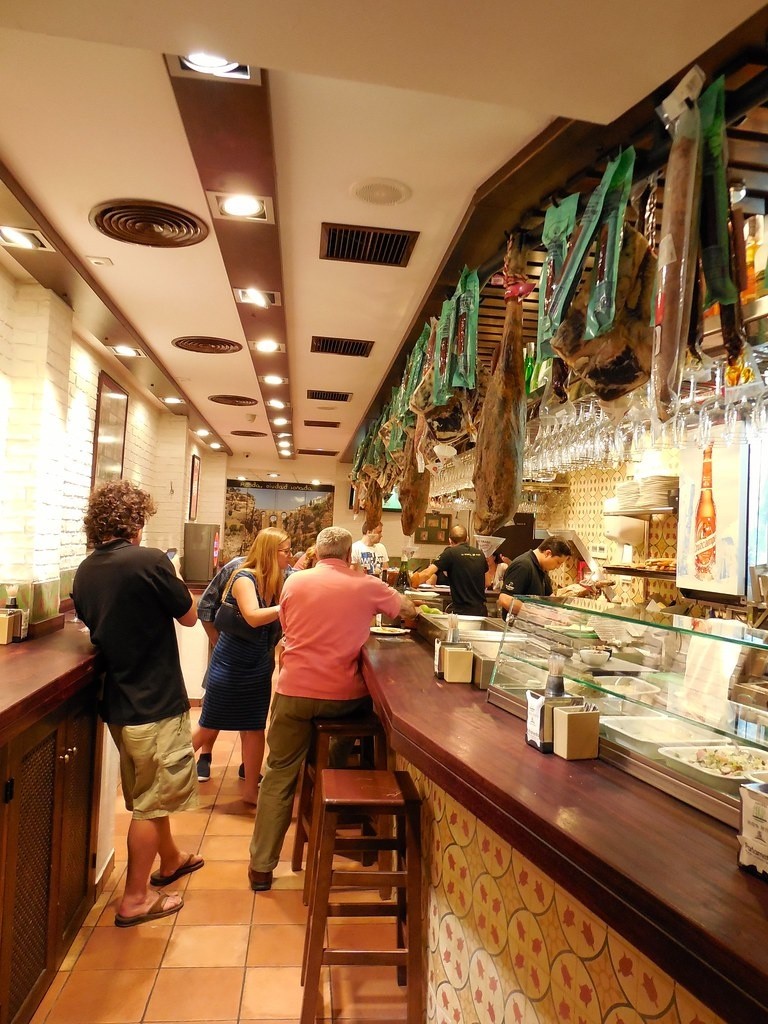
[193,513,573,890]
[72,481,205,928]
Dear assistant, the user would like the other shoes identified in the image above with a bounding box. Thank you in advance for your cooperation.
[247,867,273,891]
[196,753,212,781]
[238,763,265,787]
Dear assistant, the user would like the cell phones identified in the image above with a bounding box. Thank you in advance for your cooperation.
[164,547,178,560]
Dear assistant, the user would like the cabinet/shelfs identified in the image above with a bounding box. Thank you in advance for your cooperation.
[0,619,105,1024]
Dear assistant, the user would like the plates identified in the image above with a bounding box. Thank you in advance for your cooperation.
[405,593,440,597]
[369,626,407,634]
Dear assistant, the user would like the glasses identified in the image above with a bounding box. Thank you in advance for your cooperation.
[278,547,292,555]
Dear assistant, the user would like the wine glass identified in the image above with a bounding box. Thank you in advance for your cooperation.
[428,447,475,512]
[520,343,768,482]
[66,579,90,632]
[516,489,551,514]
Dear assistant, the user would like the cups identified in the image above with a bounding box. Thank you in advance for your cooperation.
[545,675,565,699]
[444,628,460,643]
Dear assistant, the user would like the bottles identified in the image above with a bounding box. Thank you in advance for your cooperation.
[694,443,718,582]
[358,552,411,627]
[5,597,19,609]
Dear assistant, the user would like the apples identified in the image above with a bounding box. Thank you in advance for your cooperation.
[419,604,442,615]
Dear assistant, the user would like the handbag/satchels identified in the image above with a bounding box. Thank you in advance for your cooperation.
[213,574,261,647]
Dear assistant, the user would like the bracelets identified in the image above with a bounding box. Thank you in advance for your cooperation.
[276,605,280,618]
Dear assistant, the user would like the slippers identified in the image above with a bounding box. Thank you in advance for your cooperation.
[114,890,184,926]
[150,854,204,886]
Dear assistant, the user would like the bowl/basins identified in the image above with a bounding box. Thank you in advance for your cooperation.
[579,650,610,665]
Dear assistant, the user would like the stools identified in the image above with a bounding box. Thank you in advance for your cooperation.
[291,710,383,870]
[299,769,426,1024]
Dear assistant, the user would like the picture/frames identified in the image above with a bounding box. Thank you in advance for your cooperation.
[417,516,426,529]
[437,530,445,542]
[427,518,439,528]
[418,531,428,541]
[440,516,449,530]
